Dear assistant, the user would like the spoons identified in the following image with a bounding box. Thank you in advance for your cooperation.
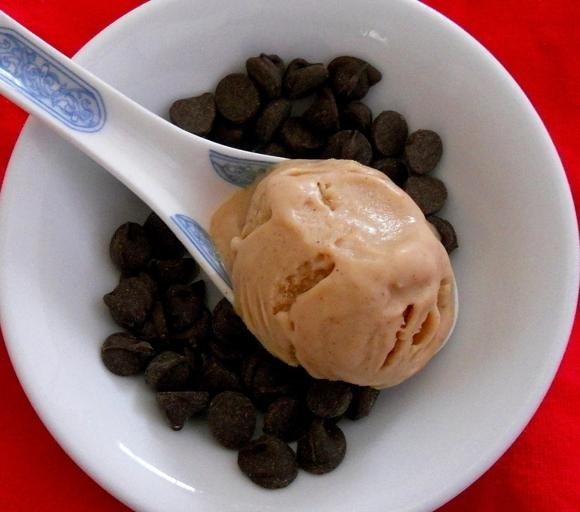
[0,11,458,369]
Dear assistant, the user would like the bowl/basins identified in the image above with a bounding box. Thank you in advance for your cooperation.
[0,1,579,511]
